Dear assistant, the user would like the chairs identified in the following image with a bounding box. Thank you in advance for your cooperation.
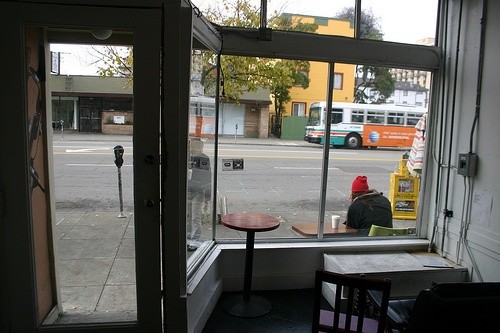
[313,269,390,333]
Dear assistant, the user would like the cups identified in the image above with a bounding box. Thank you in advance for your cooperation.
[332,215,340,228]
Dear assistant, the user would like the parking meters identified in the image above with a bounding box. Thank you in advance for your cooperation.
[113,145,127,218]
[60,121,65,139]
[234,124,239,143]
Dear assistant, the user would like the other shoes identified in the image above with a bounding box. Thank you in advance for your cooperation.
[187,240,199,250]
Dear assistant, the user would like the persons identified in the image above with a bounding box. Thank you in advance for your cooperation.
[188,140,211,250]
[346,176,393,237]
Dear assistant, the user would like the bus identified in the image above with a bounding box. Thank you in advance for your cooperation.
[304,101,428,150]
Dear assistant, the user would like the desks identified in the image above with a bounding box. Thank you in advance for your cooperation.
[292,222,356,238]
[222,213,280,318]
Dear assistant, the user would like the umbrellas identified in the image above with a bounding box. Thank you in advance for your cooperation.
[406,114,428,179]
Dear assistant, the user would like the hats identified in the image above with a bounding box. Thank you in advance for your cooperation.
[351,175,369,192]
